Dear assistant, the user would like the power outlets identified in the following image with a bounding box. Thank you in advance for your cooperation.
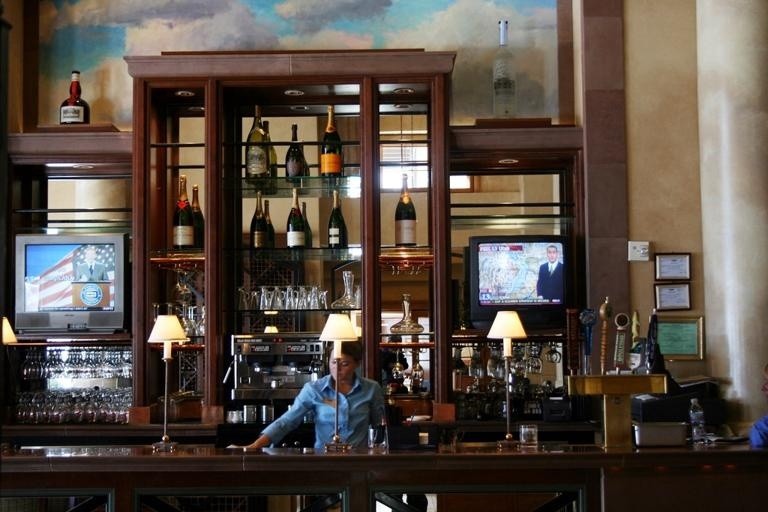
[626,242,649,267]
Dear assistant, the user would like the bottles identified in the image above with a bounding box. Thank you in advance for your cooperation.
[630,339,665,376]
[387,347,424,393]
[228,404,274,422]
[491,20,517,119]
[391,294,424,333]
[689,399,706,442]
[369,424,383,446]
[173,105,417,247]
[60,72,89,125]
[260,269,361,309]
[148,295,205,347]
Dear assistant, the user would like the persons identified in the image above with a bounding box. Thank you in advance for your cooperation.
[75,247,109,281]
[245,342,386,453]
[749,364,768,448]
[536,245,563,302]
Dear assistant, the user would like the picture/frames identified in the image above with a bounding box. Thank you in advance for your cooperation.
[648,314,707,361]
[655,283,695,312]
[653,252,692,280]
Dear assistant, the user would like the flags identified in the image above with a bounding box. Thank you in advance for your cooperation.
[31,244,115,309]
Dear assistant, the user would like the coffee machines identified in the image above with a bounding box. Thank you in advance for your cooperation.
[228,331,330,403]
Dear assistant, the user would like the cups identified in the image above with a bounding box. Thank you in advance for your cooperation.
[408,416,431,446]
[521,425,537,446]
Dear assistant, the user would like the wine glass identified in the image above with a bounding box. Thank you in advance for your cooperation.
[14,347,132,425]
[452,340,561,378]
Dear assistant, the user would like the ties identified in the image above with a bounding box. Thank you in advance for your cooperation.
[89,265,93,275]
[550,265,554,276]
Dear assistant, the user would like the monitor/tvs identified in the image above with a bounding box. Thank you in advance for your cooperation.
[14,232,129,338]
[468,235,572,331]
